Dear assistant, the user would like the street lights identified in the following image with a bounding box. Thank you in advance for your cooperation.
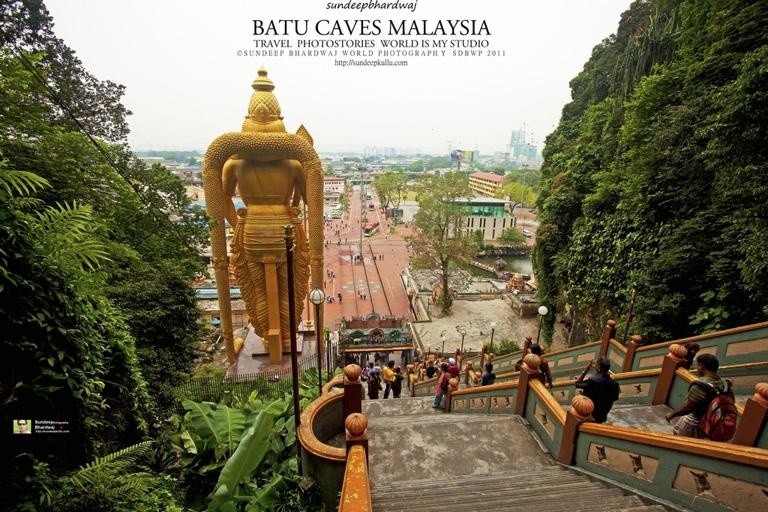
[518,162,530,235]
[535,303,547,351]
[308,285,325,396]
[426,323,499,373]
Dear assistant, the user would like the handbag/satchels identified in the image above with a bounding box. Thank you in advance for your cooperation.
[440,373,448,391]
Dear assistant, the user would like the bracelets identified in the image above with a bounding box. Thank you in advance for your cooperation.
[581,370,586,375]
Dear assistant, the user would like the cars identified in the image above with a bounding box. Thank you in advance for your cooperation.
[522,228,532,239]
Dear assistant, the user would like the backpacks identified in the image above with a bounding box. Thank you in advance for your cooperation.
[687,378,737,442]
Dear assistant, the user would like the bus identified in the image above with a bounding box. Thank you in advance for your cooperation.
[364,222,380,237]
[366,191,371,200]
[367,201,375,211]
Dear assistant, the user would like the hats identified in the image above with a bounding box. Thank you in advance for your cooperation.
[448,358,455,365]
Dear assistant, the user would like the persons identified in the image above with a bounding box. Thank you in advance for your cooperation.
[15,419,31,433]
[325,209,384,303]
[346,342,498,409]
[513,343,553,389]
[573,356,620,424]
[221,68,307,348]
[664,353,736,439]
